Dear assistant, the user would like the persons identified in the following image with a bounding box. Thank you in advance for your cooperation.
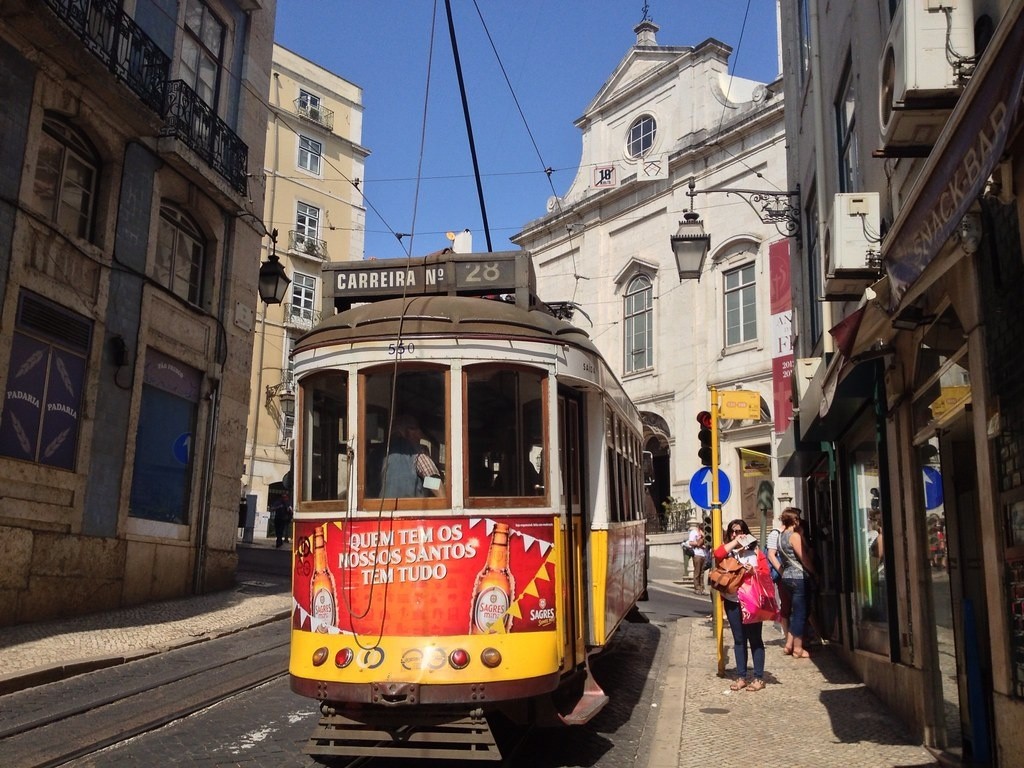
[269,494,292,548]
[764,523,787,640]
[776,510,817,658]
[709,519,777,692]
[688,523,711,595]
[366,415,446,498]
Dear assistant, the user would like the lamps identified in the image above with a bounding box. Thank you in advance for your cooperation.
[265,380,297,420]
[891,303,939,331]
[669,176,802,284]
[258,226,292,307]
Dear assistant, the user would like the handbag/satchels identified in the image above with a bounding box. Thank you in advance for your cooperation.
[764,547,784,582]
[737,566,777,624]
[686,550,694,557]
[806,575,817,593]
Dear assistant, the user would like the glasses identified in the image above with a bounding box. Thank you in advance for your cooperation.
[728,529,743,537]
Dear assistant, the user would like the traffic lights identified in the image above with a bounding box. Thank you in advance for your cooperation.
[697,410,721,466]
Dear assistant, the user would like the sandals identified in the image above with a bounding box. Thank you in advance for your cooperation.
[746,678,766,691]
[730,677,748,691]
[793,648,815,658]
[783,646,793,655]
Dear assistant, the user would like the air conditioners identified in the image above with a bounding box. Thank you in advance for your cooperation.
[820,192,886,300]
[871,0,976,159]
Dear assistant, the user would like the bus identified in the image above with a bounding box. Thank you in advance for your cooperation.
[286,250,649,764]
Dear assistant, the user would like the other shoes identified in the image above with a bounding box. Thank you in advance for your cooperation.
[702,590,709,596]
[810,637,829,646]
[695,589,702,595]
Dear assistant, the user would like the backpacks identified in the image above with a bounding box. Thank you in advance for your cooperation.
[709,557,746,594]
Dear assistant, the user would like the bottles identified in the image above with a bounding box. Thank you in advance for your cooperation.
[310,527,339,628]
[468,522,515,635]
